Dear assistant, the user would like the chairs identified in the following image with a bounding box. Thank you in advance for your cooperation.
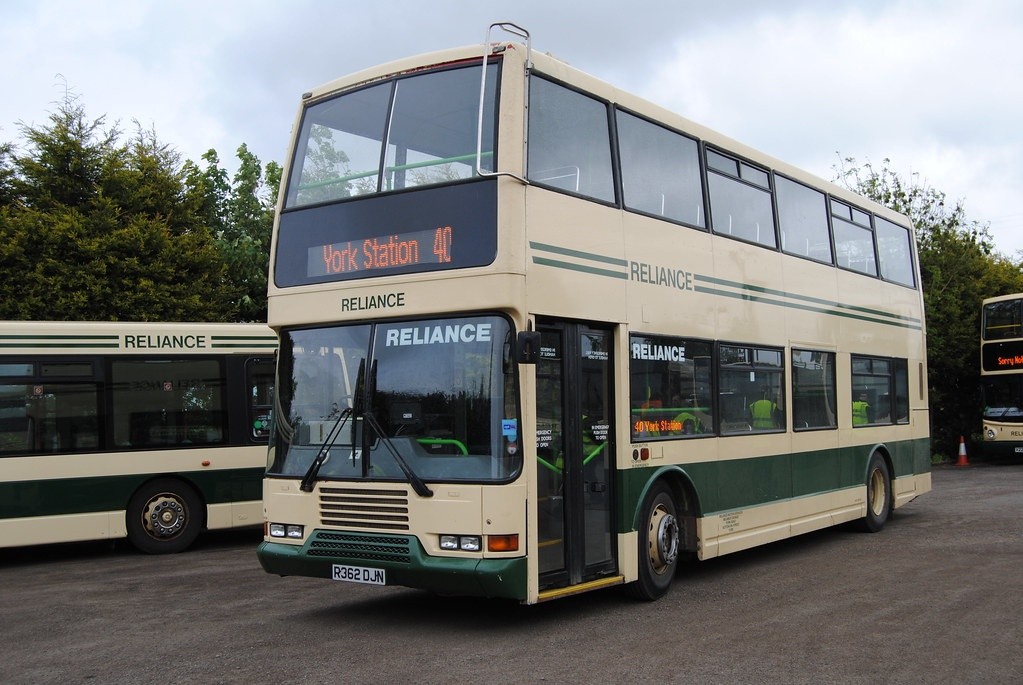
[721,421,753,432]
[529,165,580,194]
[630,192,889,278]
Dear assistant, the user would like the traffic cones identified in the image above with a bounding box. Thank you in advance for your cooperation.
[955,435,971,467]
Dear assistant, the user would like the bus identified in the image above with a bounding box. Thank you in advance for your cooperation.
[980,291,1023,456]
[253,19,931,607]
[0,317,359,554]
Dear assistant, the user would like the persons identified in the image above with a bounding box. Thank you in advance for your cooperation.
[667,399,703,435]
[639,409,661,437]
[852,392,873,424]
[746,385,780,430]
